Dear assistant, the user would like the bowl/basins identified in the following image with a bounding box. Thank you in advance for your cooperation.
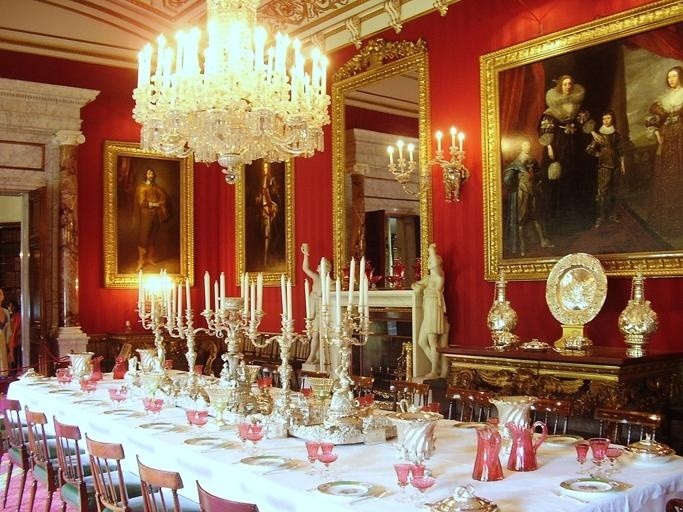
[623,448,676,464]
[16,376,44,382]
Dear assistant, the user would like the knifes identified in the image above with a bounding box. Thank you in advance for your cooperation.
[590,474,633,489]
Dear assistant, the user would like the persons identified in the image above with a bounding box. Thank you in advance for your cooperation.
[537,74,603,239]
[300,241,335,374]
[409,242,453,381]
[6,299,22,368]
[254,162,282,265]
[643,65,683,237]
[504,135,556,258]
[0,290,14,380]
[134,167,172,273]
[584,109,629,231]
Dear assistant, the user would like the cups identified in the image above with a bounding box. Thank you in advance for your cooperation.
[67,352,95,381]
[135,348,167,373]
[488,395,536,439]
[386,412,443,463]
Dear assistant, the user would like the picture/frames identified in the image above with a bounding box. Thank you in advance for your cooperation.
[234,156,296,287]
[478,0,682,281]
[101,139,194,285]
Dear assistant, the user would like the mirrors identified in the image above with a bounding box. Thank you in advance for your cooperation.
[331,35,435,292]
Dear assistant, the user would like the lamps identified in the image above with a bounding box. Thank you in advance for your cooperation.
[130,0,331,187]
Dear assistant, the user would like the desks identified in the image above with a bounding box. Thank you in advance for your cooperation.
[8,367,683,511]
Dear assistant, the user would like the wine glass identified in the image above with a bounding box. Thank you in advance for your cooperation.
[342,263,383,290]
[573,436,622,480]
[385,257,421,290]
[55,355,440,510]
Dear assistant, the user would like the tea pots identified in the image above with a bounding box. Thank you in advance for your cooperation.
[471,423,501,483]
[503,419,548,471]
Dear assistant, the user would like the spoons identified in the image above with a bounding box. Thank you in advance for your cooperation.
[551,489,590,505]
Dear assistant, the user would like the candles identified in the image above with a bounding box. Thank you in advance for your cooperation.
[134,255,371,329]
[406,142,416,162]
[394,139,405,158]
[435,129,444,150]
[449,127,456,146]
[457,131,465,151]
[385,145,394,164]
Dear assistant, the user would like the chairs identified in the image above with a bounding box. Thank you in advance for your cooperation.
[52,414,160,512]
[594,407,663,445]
[104,331,374,406]
[529,398,572,434]
[389,379,430,411]
[1,397,84,511]
[24,404,117,512]
[445,386,496,422]
[196,480,258,512]
[84,433,201,512]
[136,454,184,512]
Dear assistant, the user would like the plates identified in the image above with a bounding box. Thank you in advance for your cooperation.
[545,252,609,326]
[451,419,486,430]
[559,478,629,495]
[532,435,584,448]
[49,390,78,396]
